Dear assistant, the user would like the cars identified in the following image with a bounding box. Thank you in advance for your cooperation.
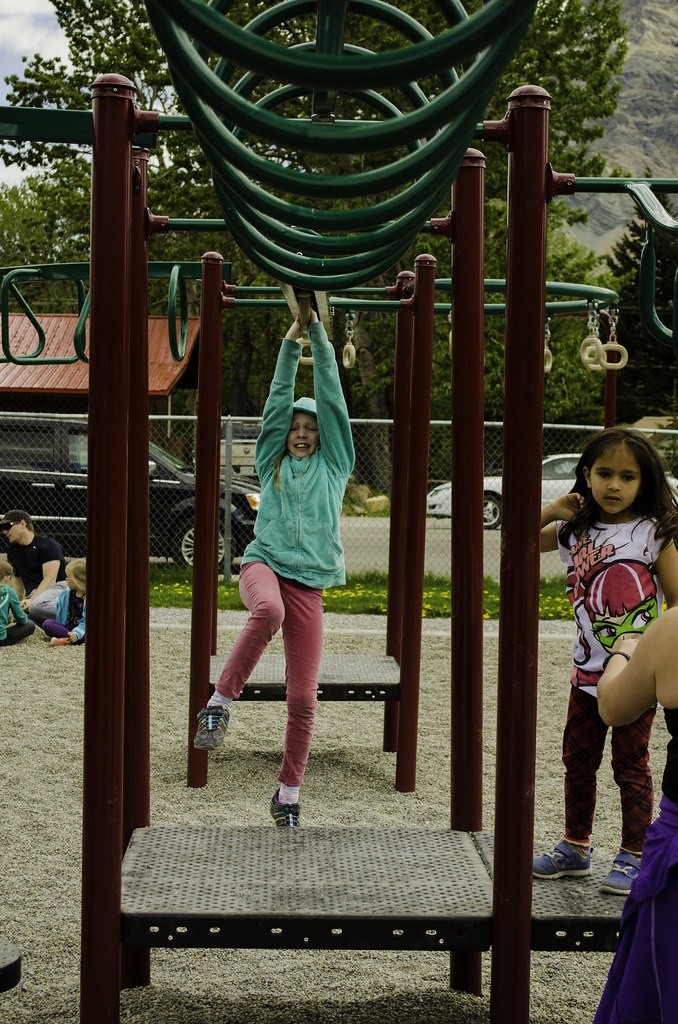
[0,409,265,572]
[425,453,678,530]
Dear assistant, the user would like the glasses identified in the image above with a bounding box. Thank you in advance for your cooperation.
[2,521,20,531]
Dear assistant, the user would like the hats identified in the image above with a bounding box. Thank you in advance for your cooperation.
[0,509,33,523]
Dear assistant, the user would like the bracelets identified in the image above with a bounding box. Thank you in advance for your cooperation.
[603,652,631,671]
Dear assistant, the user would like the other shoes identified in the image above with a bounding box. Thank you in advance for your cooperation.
[50,637,70,646]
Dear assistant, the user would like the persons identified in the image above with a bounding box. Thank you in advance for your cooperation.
[590,607,678,1024]
[530,428,678,898]
[0,510,66,628]
[193,313,355,829]
[43,556,86,646]
[0,560,36,647]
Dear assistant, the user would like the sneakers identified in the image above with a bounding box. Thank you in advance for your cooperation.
[533,837,594,879]
[599,851,642,895]
[268,789,301,827]
[193,704,230,751]
